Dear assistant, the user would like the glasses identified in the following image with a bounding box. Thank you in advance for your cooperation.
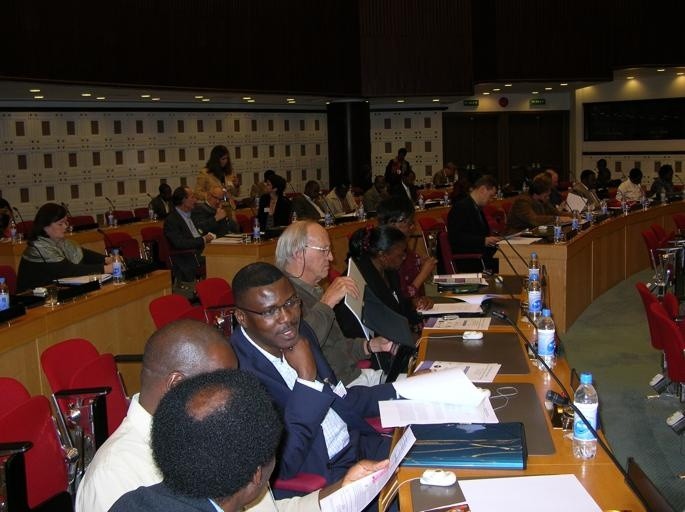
[234,297,301,320]
[305,245,334,256]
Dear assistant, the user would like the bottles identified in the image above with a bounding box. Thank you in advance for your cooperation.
[533,307,556,371]
[107,206,114,229]
[527,272,544,325]
[8,221,17,244]
[147,203,153,221]
[660,187,666,203]
[552,216,562,245]
[110,249,125,287]
[619,191,627,209]
[217,166,521,253]
[0,278,10,313]
[571,371,599,460]
[570,209,579,231]
[600,199,607,215]
[528,251,541,282]
[63,213,69,234]
[584,202,592,224]
[640,189,647,209]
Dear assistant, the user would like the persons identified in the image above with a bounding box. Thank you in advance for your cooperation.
[364,148,419,213]
[510,168,573,230]
[194,145,239,233]
[276,220,399,385]
[152,183,173,220]
[0,199,14,243]
[164,185,214,282]
[15,203,126,302]
[326,177,359,214]
[433,162,501,273]
[250,170,275,204]
[106,368,284,512]
[293,180,330,223]
[259,174,293,236]
[227,261,403,512]
[191,185,235,238]
[571,158,611,208]
[343,199,438,343]
[616,164,672,201]
[75,318,399,512]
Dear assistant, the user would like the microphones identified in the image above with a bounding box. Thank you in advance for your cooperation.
[478,267,564,356]
[484,241,548,308]
[24,237,71,300]
[316,190,343,226]
[15,204,29,238]
[544,390,656,512]
[675,173,684,197]
[489,311,599,430]
[243,200,268,241]
[491,222,547,283]
[286,179,307,202]
[550,187,604,228]
[99,190,121,227]
[146,190,165,218]
